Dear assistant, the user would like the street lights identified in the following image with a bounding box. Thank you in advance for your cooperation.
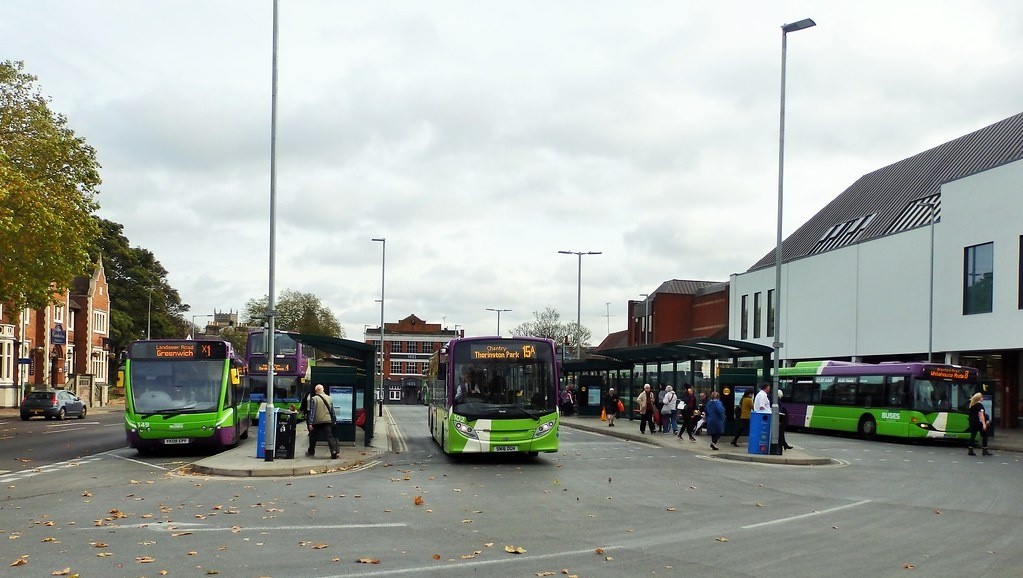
[371,238,385,418]
[454,325,462,337]
[192,315,214,339]
[639,294,648,345]
[557,251,602,359]
[916,202,936,363]
[487,308,512,335]
[768,19,816,453]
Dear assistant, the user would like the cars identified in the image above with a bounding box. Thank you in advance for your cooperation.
[21,390,87,421]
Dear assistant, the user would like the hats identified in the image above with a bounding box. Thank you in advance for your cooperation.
[664,385,672,392]
[778,389,783,397]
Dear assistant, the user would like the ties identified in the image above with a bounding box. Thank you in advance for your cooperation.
[468,384,469,392]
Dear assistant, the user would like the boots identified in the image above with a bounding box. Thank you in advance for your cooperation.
[968,447,976,455]
[982,447,993,455]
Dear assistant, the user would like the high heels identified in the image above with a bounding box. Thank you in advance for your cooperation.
[784,445,793,450]
[731,439,739,447]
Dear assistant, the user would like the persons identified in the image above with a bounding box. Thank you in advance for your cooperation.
[455,374,482,402]
[637,383,793,450]
[299,384,337,459]
[558,383,578,417]
[603,387,619,427]
[968,393,993,456]
[417,391,422,404]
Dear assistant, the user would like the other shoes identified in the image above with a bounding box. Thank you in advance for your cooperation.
[688,437,695,441]
[611,424,614,426]
[709,444,718,450]
[331,453,338,459]
[609,424,611,427]
[651,430,656,434]
[677,435,683,440]
[305,452,314,457]
[673,431,678,435]
[641,431,644,434]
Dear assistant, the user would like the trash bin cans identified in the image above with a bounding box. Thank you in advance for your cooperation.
[256,407,280,459]
[748,411,772,455]
[770,412,786,456]
[274,409,299,459]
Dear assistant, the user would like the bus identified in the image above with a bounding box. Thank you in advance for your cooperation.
[756,361,989,440]
[427,336,561,459]
[116,340,251,456]
[246,329,315,425]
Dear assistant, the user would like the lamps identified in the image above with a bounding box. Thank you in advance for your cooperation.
[91,351,101,360]
[106,353,116,361]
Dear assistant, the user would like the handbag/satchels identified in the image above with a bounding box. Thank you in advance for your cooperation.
[735,405,741,418]
[660,405,671,415]
[617,400,624,412]
[602,407,606,421]
